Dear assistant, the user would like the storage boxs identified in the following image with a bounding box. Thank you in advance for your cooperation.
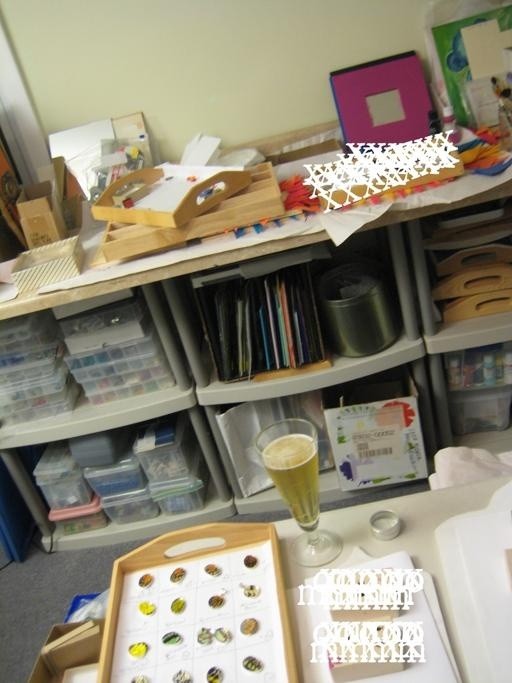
[2,291,189,438]
[16,179,69,250]
[448,392,511,436]
[445,341,512,391]
[29,594,105,683]
[320,369,428,493]
[1,411,209,531]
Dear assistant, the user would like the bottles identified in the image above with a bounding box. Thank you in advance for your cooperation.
[442,106,459,143]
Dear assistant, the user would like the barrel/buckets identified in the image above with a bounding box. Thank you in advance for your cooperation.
[313,259,399,356]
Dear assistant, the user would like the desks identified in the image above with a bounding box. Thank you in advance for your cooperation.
[269,479,512,683]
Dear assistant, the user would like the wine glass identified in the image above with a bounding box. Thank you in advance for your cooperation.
[254,416,343,568]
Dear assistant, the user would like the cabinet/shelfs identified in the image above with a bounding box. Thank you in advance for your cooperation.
[0,147,512,552]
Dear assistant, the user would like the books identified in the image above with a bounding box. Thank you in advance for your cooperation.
[39,620,103,683]
[212,271,332,385]
